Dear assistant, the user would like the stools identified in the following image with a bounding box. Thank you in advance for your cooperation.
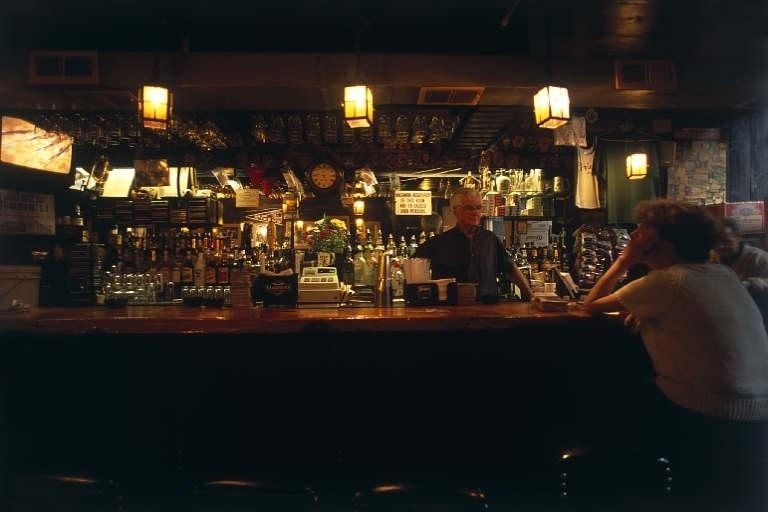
[29,444,675,512]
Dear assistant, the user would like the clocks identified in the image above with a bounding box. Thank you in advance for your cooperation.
[303,159,346,195]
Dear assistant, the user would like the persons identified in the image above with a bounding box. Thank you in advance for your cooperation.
[708,215,768,295]
[584,199,767,423]
[408,186,546,304]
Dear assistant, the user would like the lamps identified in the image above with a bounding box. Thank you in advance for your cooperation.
[625,152,649,184]
[136,81,176,134]
[532,85,572,131]
[352,199,368,217]
[340,81,375,131]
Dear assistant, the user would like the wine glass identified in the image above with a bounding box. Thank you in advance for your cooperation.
[36,113,227,151]
[252,114,460,146]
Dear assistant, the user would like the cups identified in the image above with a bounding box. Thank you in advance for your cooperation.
[317,250,335,267]
[95,265,232,307]
[7,296,24,313]
[545,283,556,294]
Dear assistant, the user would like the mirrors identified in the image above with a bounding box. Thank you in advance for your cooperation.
[65,149,575,288]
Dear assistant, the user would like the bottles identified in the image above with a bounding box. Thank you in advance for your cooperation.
[342,228,435,308]
[434,168,540,195]
[115,230,247,285]
[518,226,568,264]
[57,204,88,243]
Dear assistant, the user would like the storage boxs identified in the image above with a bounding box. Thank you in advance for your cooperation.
[1,264,43,312]
[1,188,58,238]
[706,200,767,236]
